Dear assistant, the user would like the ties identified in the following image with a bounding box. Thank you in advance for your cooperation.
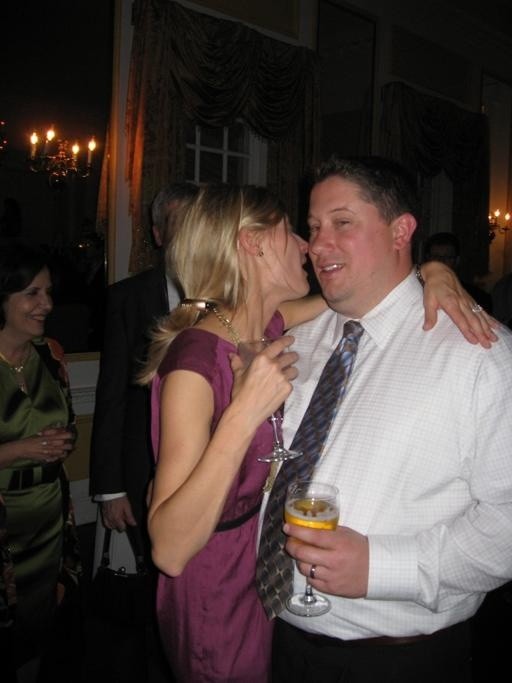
[255,319,365,621]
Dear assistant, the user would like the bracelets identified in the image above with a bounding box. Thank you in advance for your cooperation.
[416,263,425,287]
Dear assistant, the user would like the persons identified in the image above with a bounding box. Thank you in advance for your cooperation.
[421,230,459,269]
[0,254,79,682]
[254,154,511,683]
[88,178,205,682]
[137,182,505,682]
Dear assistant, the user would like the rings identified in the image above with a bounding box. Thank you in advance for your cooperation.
[309,565,318,580]
[474,303,484,313]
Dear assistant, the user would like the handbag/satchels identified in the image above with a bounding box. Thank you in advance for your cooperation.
[87,523,164,658]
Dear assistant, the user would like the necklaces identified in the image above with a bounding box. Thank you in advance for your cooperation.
[211,307,244,350]
[11,342,31,374]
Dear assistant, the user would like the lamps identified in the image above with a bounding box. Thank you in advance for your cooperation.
[489,208,510,242]
[28,123,98,189]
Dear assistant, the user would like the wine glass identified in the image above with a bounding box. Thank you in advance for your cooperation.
[236,340,304,465]
[283,481,341,619]
